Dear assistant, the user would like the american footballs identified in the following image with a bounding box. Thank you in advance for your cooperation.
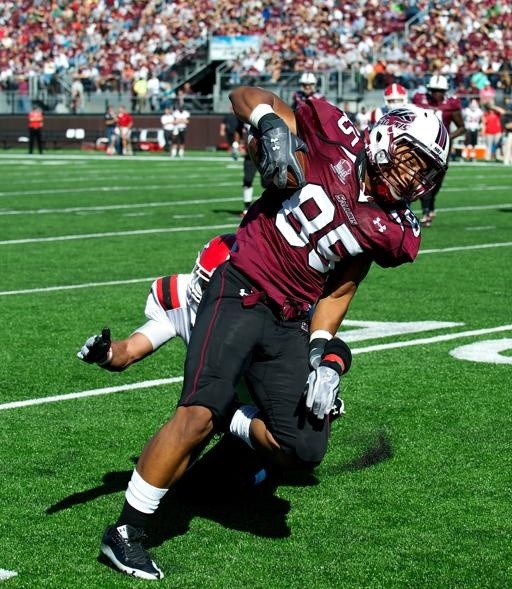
[248,125,308,190]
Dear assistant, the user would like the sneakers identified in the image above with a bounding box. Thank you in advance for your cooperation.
[100,525,165,580]
[189,397,236,466]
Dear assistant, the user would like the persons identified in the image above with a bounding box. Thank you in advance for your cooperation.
[238,133,257,222]
[99,83,450,583]
[77,235,356,508]
[1,0,512,165]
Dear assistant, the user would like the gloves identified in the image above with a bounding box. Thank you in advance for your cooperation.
[307,337,352,421]
[250,104,308,190]
[77,327,114,365]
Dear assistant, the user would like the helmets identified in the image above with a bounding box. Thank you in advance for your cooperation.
[188,234,235,313]
[384,84,405,108]
[430,75,447,91]
[299,73,317,84]
[365,108,449,207]
